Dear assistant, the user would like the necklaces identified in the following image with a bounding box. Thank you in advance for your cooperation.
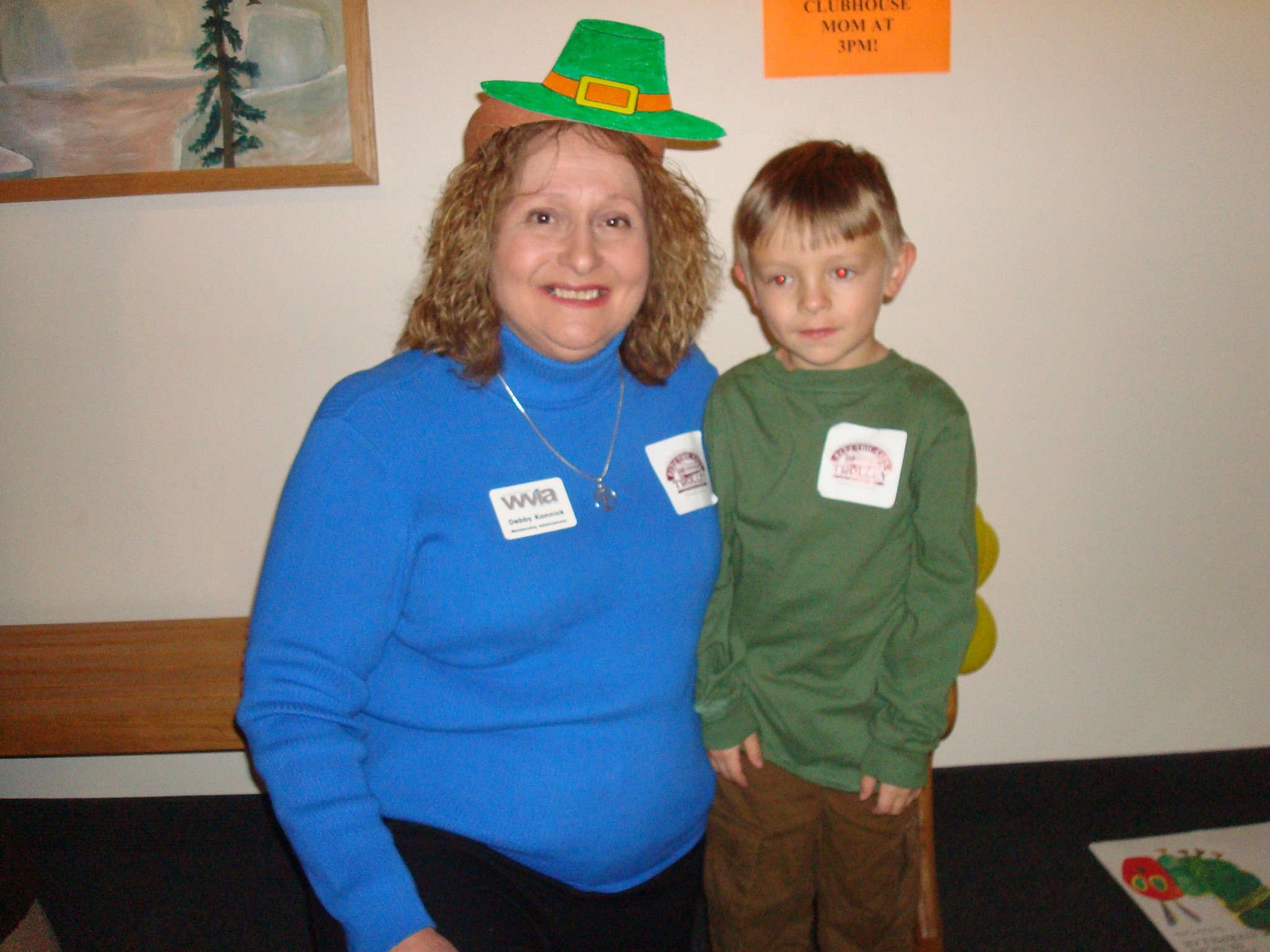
[497,351,625,510]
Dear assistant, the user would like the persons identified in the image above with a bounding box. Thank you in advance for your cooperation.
[693,140,980,952]
[235,119,721,952]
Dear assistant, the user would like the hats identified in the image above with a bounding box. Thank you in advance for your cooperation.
[482,19,725,141]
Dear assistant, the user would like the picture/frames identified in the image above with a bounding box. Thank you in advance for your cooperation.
[0,0,378,202]
